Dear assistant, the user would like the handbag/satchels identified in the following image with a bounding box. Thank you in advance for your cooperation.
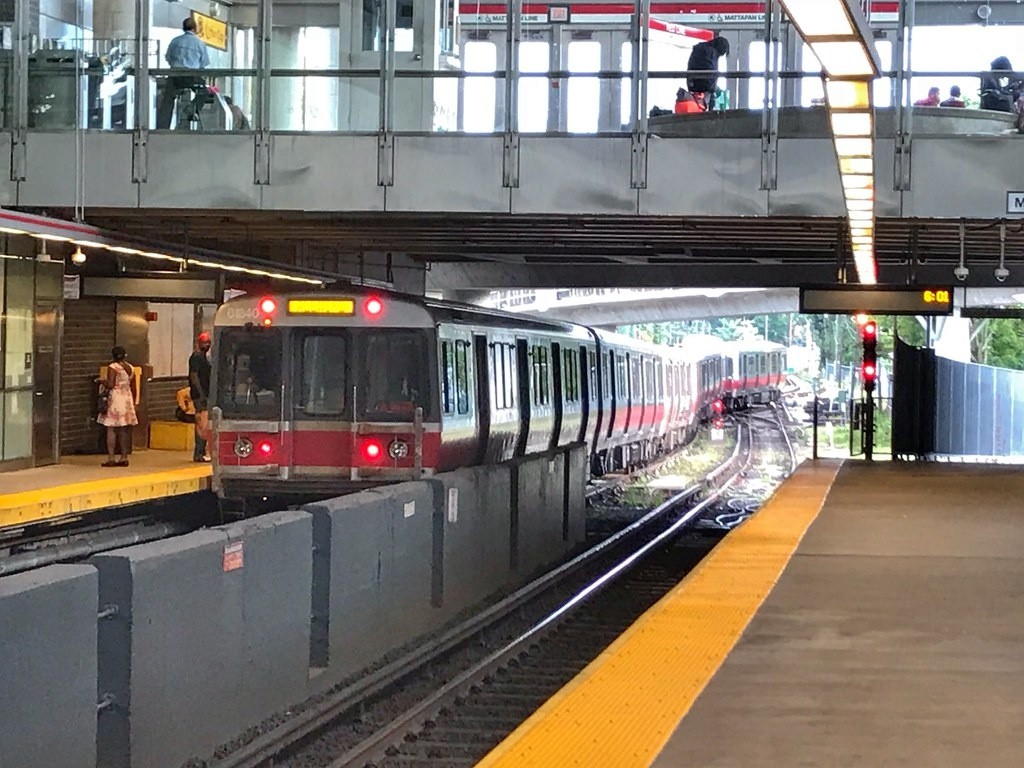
[196,404,208,440]
[97,391,108,415]
[676,92,708,113]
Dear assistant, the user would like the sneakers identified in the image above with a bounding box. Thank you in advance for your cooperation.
[101,460,117,467]
[115,460,129,467]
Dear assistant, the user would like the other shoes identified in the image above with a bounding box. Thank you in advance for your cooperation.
[183,105,199,121]
[194,456,212,462]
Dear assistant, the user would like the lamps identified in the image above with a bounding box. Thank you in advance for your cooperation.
[954,224,969,280]
[977,4,991,19]
[70,246,87,263]
[37,240,50,260]
[995,225,1009,282]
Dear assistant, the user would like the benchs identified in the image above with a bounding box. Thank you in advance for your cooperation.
[150,420,195,451]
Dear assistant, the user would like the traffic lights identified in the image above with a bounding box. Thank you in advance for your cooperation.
[862,322,877,387]
[710,398,724,424]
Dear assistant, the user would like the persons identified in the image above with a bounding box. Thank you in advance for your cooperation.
[188,333,211,463]
[157,17,209,129]
[940,85,966,107]
[95,346,138,468]
[686,37,730,110]
[914,87,940,106]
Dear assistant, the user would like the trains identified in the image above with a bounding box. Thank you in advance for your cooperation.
[207,288,789,504]
